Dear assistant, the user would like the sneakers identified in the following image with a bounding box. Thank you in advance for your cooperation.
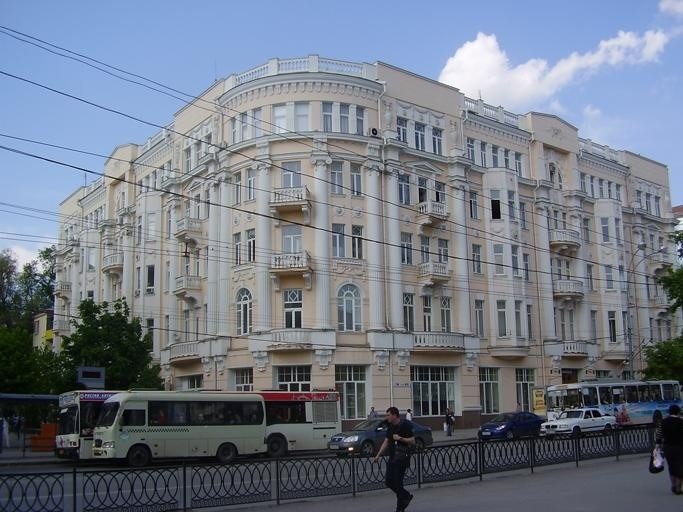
[670,486,681,493]
[395,493,414,512]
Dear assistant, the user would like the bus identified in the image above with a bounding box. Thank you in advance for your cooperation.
[544,380,680,427]
[56,388,341,466]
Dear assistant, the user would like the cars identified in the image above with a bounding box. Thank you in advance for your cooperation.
[539,409,616,436]
[328,416,433,455]
[477,411,546,439]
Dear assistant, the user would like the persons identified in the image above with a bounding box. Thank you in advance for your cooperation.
[369,405,415,512]
[368,406,377,419]
[653,404,683,494]
[405,409,412,421]
[157,408,259,424]
[445,407,452,436]
[613,407,623,425]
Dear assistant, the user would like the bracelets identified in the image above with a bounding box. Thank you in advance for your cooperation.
[399,437,403,441]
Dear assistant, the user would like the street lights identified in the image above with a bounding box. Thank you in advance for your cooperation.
[625,241,668,379]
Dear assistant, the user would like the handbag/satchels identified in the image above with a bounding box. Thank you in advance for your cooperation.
[391,451,410,467]
[649,453,664,473]
